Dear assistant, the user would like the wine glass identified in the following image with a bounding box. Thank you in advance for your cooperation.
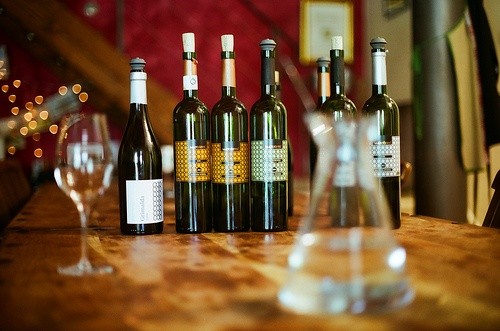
[53,111,115,276]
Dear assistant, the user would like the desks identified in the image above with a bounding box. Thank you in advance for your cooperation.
[0,174,500,331]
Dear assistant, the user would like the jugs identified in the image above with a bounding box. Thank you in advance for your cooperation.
[275,109,414,314]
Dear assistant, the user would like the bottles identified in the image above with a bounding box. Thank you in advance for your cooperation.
[211,34,251,233]
[117,58,164,235]
[310,35,360,228]
[358,36,402,230]
[249,39,293,232]
[172,32,214,234]
